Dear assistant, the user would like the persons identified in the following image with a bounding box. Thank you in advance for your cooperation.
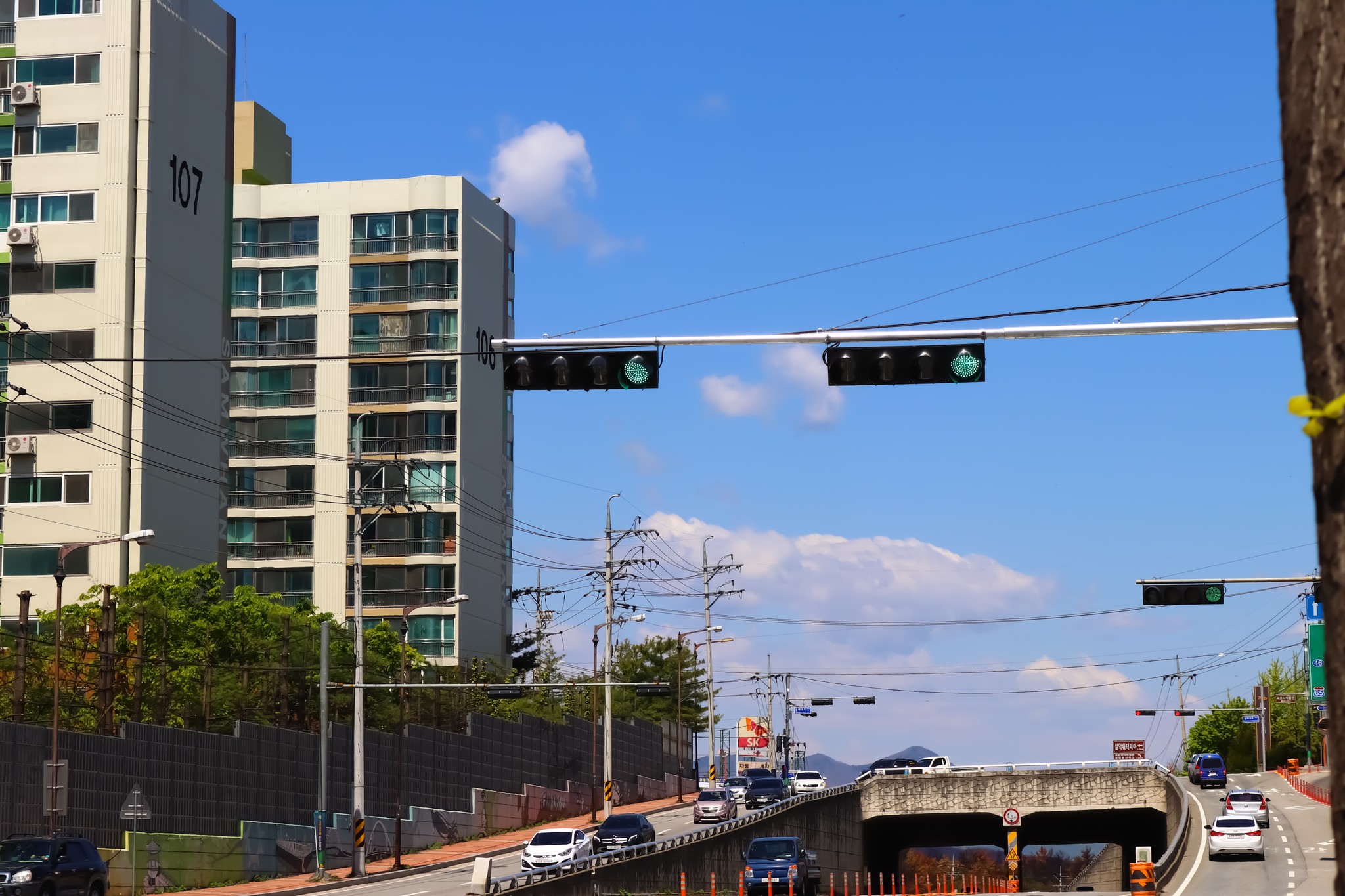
[31,844,51,860]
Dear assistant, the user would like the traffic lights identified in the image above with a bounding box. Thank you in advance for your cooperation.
[1175,711,1195,716]
[827,342,986,387]
[502,349,660,391]
[784,737,791,753]
[1135,711,1155,716]
[1303,713,1311,731]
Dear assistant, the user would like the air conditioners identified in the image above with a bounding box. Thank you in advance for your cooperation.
[10,81,38,106]
[5,225,35,248]
[5,433,35,457]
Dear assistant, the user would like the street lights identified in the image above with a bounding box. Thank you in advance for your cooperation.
[677,624,723,803]
[693,637,734,790]
[393,594,471,872]
[590,614,647,822]
[49,528,156,836]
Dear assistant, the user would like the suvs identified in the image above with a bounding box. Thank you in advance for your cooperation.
[692,787,738,824]
[1185,752,1227,789]
[0,831,110,896]
[859,758,923,783]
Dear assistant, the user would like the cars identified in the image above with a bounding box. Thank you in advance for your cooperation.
[1075,886,1094,892]
[719,768,791,810]
[960,886,980,893]
[521,828,593,870]
[1204,790,1271,861]
[591,814,655,855]
[790,771,827,796]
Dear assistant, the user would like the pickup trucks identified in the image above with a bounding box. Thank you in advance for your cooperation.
[917,756,985,774]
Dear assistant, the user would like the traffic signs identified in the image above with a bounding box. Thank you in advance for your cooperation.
[1316,705,1328,711]
[1276,695,1296,703]
[1112,740,1145,760]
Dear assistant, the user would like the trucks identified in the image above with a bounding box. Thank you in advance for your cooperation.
[740,836,822,896]
